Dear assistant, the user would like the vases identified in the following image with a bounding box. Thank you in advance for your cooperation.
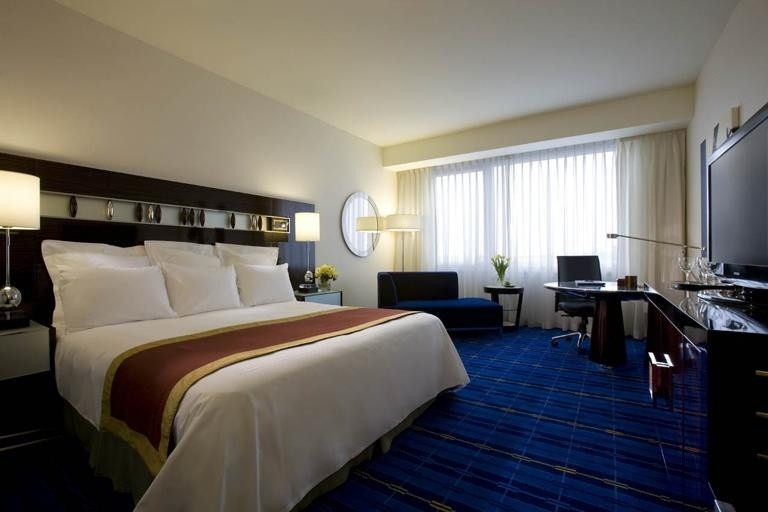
[318,280,332,292]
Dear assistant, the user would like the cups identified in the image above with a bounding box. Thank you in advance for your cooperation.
[625,276,637,290]
[617,278,625,288]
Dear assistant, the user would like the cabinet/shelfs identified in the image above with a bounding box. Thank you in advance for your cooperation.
[644,281,768,512]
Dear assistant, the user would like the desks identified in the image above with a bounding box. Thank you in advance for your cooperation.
[545,281,645,366]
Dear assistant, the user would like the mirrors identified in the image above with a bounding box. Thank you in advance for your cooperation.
[341,192,380,258]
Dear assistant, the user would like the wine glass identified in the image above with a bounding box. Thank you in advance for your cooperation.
[679,291,721,318]
[696,256,718,284]
[679,256,695,281]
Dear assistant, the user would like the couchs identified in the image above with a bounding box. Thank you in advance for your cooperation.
[378,272,505,332]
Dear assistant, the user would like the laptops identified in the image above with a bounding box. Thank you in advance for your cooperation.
[574,280,605,287]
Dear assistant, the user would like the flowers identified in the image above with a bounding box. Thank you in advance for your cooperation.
[315,264,339,281]
[491,255,511,281]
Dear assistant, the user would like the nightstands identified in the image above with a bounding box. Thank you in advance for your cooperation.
[0,321,56,456]
[294,289,342,306]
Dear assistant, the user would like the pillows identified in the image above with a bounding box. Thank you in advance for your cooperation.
[42,237,297,334]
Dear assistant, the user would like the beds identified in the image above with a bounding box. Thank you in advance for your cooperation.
[53,301,472,512]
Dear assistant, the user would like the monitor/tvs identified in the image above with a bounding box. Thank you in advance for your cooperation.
[705,101,767,290]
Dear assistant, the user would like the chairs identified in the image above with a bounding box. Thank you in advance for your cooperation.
[551,256,602,353]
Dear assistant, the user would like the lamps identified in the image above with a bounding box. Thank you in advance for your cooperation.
[0,170,41,331]
[607,233,707,251]
[357,216,377,243]
[378,214,420,273]
[294,212,321,293]
